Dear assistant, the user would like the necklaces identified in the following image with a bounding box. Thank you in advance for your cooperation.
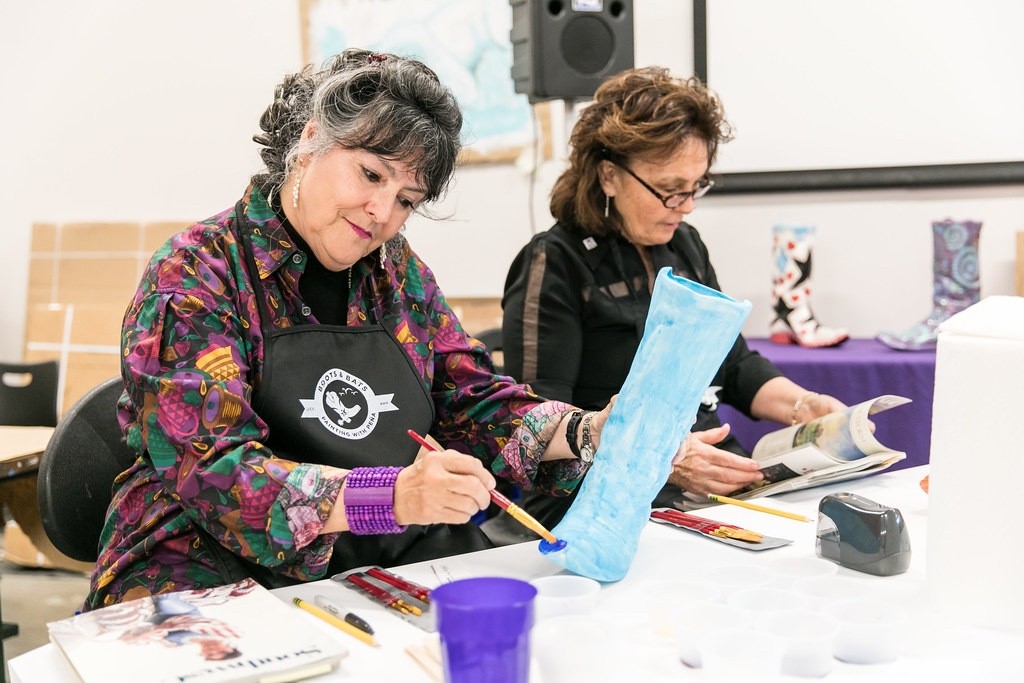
[266,183,352,290]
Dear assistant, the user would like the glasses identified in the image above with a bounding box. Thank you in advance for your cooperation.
[610,159,715,209]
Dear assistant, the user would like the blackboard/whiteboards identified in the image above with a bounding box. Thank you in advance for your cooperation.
[690,0,1024,193]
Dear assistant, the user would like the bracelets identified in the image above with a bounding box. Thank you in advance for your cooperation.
[791,391,815,426]
[345,467,409,533]
[565,410,590,458]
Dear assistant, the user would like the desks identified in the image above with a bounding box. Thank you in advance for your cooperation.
[714,334,937,474]
[0,425,57,683]
[0,460,1024,683]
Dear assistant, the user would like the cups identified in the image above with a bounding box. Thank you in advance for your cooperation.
[430,578,539,683]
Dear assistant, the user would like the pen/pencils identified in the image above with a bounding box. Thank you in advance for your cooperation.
[314,594,375,636]
[707,492,815,522]
[292,596,381,649]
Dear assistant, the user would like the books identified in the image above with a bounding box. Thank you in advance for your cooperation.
[731,395,912,495]
[45,578,349,683]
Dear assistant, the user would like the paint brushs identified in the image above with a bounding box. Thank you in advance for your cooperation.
[407,429,560,548]
[651,509,765,543]
[347,568,432,616]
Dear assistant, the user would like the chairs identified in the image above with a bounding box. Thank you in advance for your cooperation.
[0,360,150,563]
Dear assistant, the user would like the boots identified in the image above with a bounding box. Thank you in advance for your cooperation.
[877,219,983,351]
[770,224,850,350]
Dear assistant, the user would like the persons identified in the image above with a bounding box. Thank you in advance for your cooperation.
[117,591,243,661]
[81,52,620,613]
[501,71,875,499]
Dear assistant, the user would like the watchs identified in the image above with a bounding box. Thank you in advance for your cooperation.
[580,411,599,464]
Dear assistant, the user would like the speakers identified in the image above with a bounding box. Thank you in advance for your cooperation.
[507,0,634,106]
